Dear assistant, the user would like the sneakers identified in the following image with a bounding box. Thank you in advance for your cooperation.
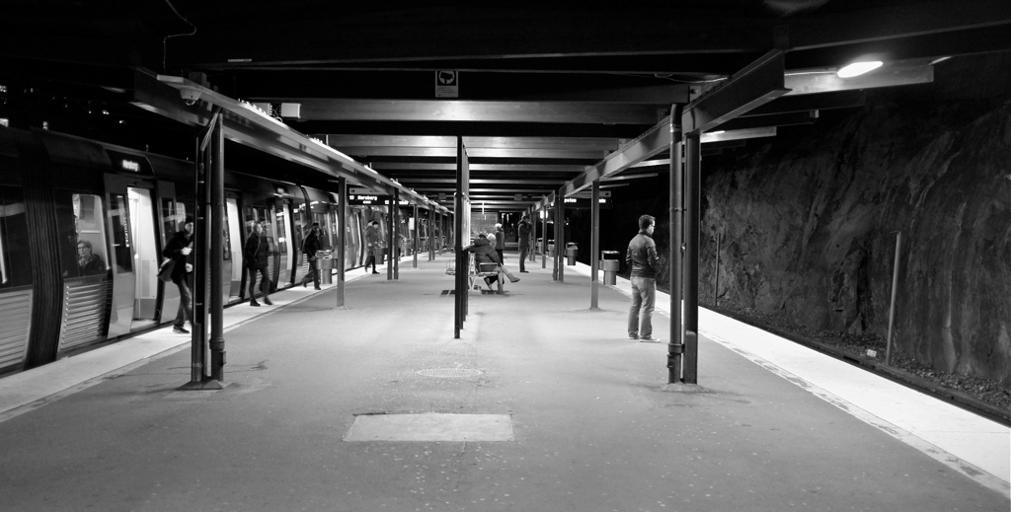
[629,333,661,342]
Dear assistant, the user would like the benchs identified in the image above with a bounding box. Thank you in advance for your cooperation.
[472,257,504,293]
[444,259,459,294]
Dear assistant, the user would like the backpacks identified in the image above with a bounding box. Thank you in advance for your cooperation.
[157,257,175,283]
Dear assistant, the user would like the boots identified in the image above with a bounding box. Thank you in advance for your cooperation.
[507,272,520,283]
[497,284,509,294]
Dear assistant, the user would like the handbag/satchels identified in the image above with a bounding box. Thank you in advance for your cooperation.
[301,239,321,254]
[259,277,277,294]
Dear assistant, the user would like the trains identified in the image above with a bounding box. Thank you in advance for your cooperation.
[0,114,446,377]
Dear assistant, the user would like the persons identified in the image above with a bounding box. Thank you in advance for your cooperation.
[625,214,660,342]
[62,240,106,278]
[161,217,194,334]
[516,215,533,273]
[223,218,520,307]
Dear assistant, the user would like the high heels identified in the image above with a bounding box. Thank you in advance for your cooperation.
[250,298,260,306]
[264,297,272,304]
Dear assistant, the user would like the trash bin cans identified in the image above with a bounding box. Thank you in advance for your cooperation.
[420,236,446,252]
[566,242,578,265]
[375,248,385,264]
[601,250,620,285]
[537,237,543,254]
[547,239,555,257]
[315,250,333,284]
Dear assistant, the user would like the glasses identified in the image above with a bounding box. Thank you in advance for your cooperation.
[651,224,655,228]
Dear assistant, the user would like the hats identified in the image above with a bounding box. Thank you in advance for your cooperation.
[184,216,193,225]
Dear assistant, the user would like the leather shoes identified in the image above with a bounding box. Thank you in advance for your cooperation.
[372,271,380,274]
[301,278,306,286]
[173,326,188,334]
[316,287,322,289]
[365,266,367,272]
